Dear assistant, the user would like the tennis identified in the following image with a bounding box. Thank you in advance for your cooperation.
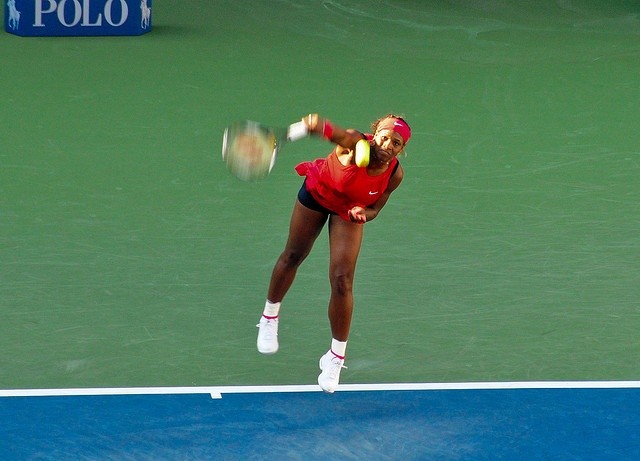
[355,140,370,168]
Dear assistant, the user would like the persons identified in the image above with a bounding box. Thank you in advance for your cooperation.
[256,114,412,395]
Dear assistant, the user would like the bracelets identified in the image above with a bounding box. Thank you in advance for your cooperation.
[322,119,333,141]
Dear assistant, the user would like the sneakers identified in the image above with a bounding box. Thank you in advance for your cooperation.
[255,315,279,355]
[318,348,347,394]
